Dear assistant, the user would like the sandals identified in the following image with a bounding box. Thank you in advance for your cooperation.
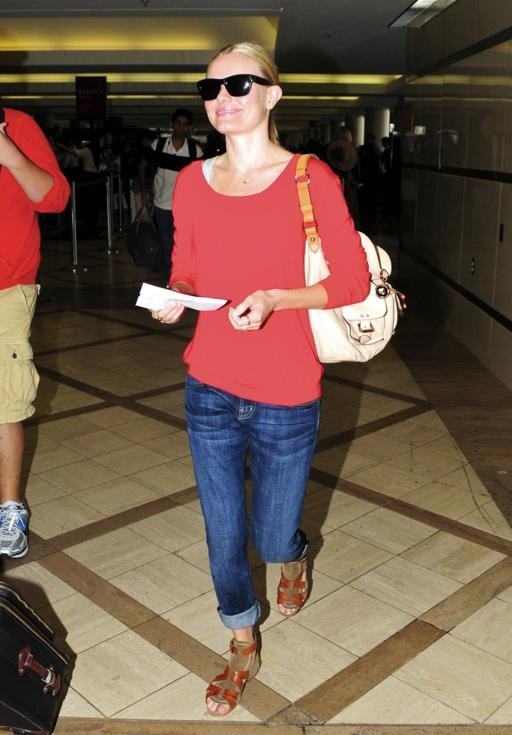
[205,633,262,716]
[276,560,308,616]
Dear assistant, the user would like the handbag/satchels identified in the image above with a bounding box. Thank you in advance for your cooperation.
[305,231,406,364]
[127,220,161,273]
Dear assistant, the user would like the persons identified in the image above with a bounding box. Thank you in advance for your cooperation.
[281,124,394,235]
[147,40,373,717]
[0,97,76,560]
[32,107,227,285]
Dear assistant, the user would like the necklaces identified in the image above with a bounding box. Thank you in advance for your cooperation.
[227,140,271,185]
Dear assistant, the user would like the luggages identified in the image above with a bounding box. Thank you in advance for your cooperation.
[0,581,75,735]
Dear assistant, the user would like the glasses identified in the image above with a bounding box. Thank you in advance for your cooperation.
[195,74,270,101]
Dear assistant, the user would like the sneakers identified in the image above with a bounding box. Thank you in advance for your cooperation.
[0,500,30,560]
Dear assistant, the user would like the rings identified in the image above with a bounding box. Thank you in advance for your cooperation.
[152,312,162,321]
[245,314,250,325]
